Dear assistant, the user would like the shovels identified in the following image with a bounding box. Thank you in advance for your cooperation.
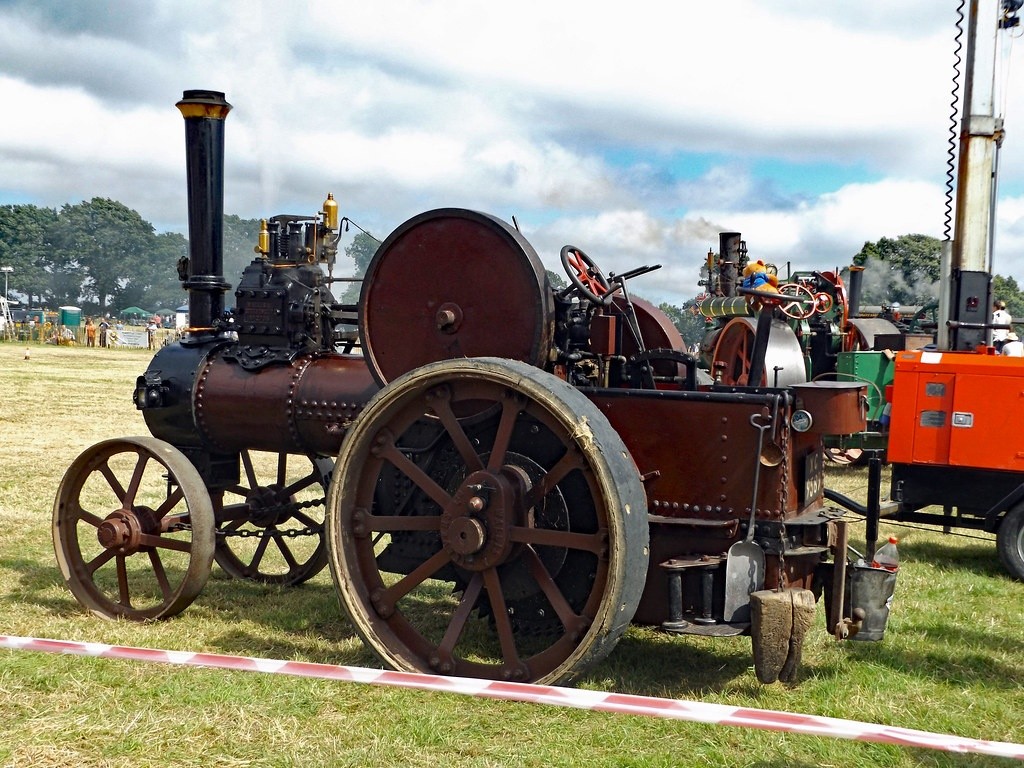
[723,414,775,624]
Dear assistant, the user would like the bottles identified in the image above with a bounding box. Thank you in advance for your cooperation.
[871,536,899,572]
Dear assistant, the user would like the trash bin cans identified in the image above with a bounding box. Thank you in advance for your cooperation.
[820,554,898,641]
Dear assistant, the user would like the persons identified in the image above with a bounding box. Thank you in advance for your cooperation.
[22,312,186,352]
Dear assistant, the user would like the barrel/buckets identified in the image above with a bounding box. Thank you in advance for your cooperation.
[821,561,898,641]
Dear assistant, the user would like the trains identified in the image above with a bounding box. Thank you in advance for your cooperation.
[41,85,906,697]
[821,0,1024,585]
[690,230,939,475]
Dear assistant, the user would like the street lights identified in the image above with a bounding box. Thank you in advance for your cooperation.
[1,265,15,300]
